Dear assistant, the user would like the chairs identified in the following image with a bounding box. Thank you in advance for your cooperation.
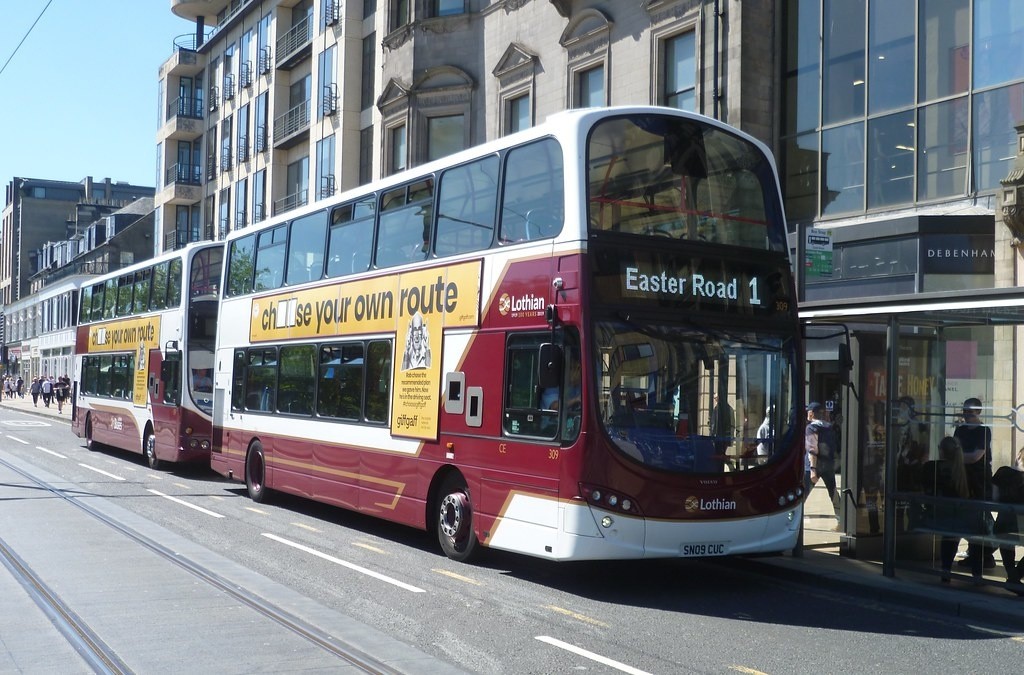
[260,386,304,414]
[252,207,708,292]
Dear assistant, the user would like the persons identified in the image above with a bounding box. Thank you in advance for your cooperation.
[3,376,25,398]
[857,426,883,537]
[804,402,841,533]
[897,425,920,464]
[937,437,974,582]
[892,396,929,532]
[708,393,736,471]
[53,376,69,414]
[63,374,71,402]
[540,359,581,431]
[954,398,997,567]
[190,364,212,392]
[991,447,1024,593]
[756,405,775,465]
[30,375,55,408]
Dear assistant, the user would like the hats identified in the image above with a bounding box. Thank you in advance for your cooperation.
[805,402,824,411]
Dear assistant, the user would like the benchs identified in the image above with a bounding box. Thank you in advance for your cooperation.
[913,491,1024,547]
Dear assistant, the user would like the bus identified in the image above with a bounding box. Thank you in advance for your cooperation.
[70,240,244,469]
[208,102,856,565]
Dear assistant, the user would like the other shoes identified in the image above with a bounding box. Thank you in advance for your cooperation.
[871,530,884,536]
[1004,578,1024,593]
[59,411,62,414]
[836,523,843,531]
[942,571,952,582]
[958,555,996,568]
